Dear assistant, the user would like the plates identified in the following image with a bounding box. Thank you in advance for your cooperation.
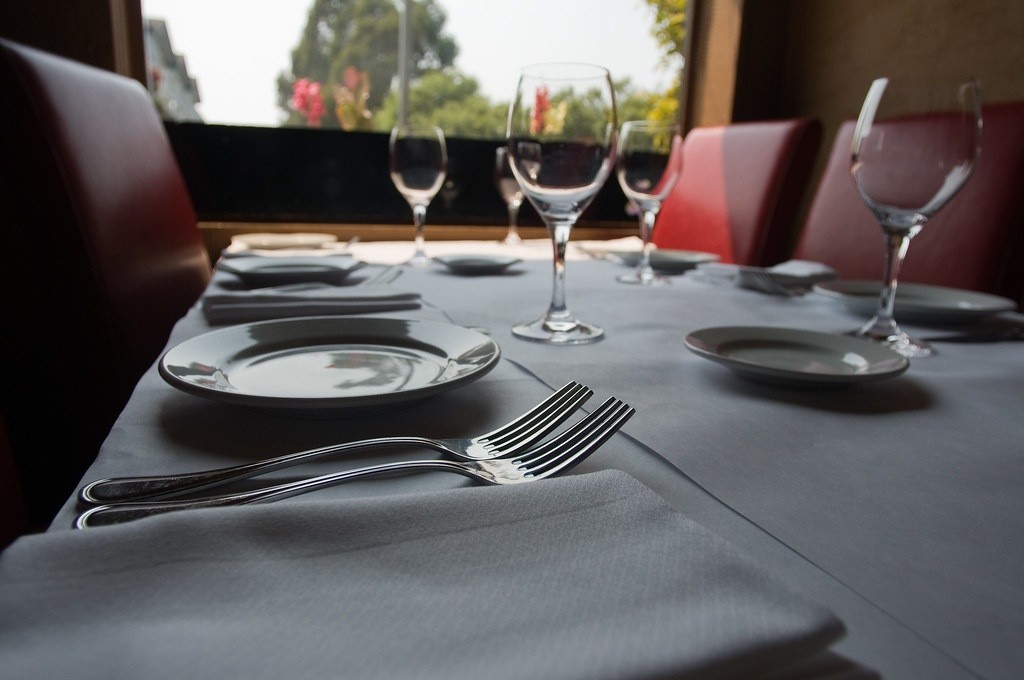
[683,325,910,392]
[233,231,340,248]
[433,252,522,276]
[215,255,369,284]
[158,316,501,409]
[618,249,720,273]
[812,278,1018,323]
[572,236,644,258]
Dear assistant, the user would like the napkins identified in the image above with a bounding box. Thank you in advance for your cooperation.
[0,466,849,680]
[202,290,422,327]
[691,258,836,296]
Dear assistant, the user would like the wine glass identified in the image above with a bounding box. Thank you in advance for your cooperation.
[507,63,617,346]
[495,143,541,248]
[388,125,448,269]
[616,120,683,286]
[843,75,986,359]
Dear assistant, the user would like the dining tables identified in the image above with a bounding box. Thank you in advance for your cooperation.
[0,229,1024,680]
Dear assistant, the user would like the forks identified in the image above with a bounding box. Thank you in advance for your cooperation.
[72,380,636,531]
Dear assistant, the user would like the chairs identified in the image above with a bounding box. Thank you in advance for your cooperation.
[0,34,212,549]
[650,119,824,269]
[794,101,1024,291]
